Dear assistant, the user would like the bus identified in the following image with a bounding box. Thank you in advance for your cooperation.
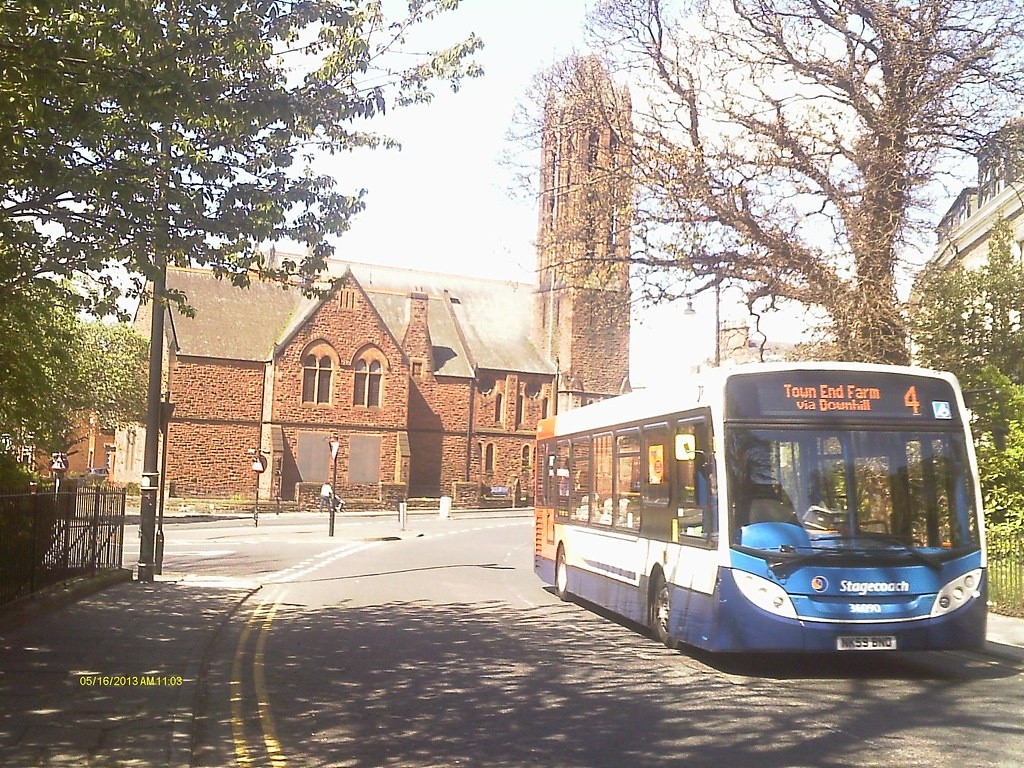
[533,359,990,670]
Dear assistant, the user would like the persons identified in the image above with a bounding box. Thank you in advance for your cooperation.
[318,481,334,515]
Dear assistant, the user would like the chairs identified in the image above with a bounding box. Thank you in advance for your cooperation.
[578,492,640,528]
